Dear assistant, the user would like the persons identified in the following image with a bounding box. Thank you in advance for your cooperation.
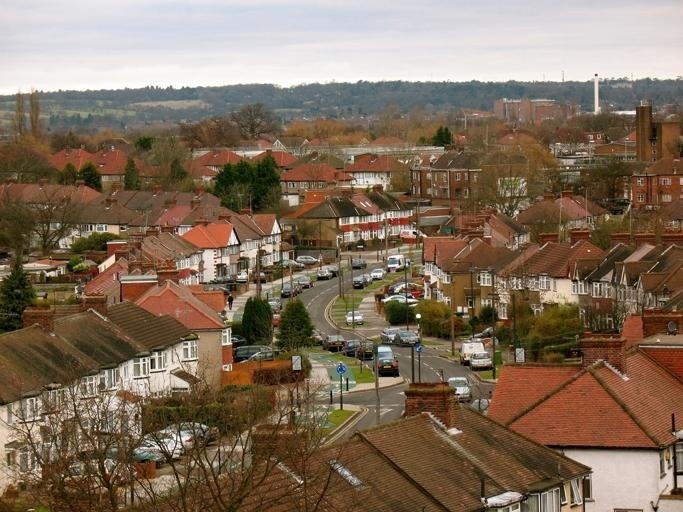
[228,294,233,310]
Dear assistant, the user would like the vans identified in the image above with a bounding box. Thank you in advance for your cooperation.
[458,340,486,366]
[398,228,427,242]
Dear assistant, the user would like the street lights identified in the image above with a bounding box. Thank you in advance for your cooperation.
[451,259,475,338]
[487,291,515,364]
[467,267,497,381]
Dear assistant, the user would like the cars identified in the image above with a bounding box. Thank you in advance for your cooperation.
[56,422,218,489]
[468,351,493,372]
[445,377,472,402]
[211,254,423,377]
